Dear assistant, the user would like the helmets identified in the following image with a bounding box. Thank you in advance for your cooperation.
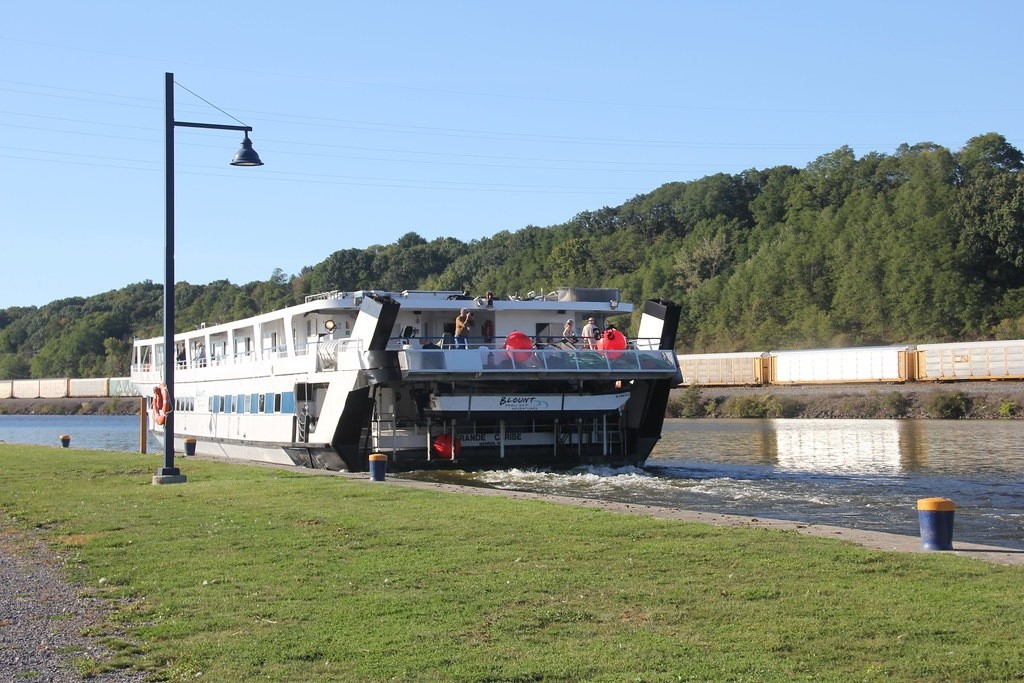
[589,317,595,321]
[567,319,574,324]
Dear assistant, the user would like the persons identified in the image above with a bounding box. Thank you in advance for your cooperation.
[582,317,598,350]
[177,343,186,369]
[196,342,206,367]
[482,320,494,343]
[529,336,554,350]
[563,319,577,343]
[454,309,471,349]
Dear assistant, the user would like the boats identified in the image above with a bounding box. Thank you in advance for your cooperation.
[129,287,684,474]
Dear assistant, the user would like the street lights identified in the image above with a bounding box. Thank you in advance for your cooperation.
[150,72,263,486]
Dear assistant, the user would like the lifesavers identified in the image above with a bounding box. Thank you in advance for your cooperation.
[483,320,493,342]
[153,382,170,426]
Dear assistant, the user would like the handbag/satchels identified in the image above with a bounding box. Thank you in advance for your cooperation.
[593,328,601,340]
